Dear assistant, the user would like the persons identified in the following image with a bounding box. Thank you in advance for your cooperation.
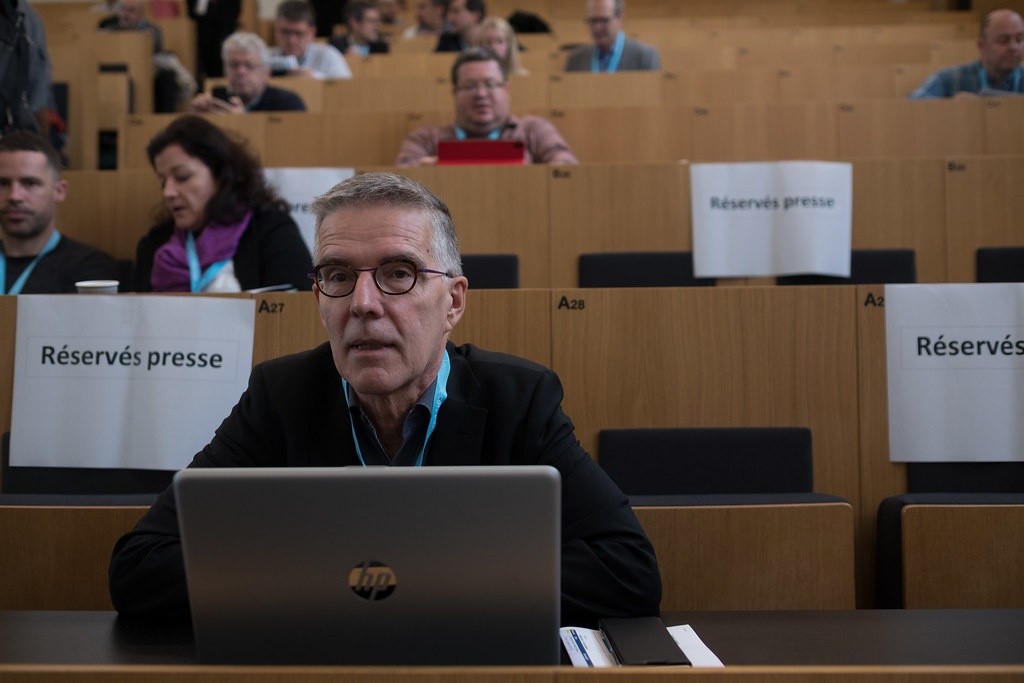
[269,0,404,79]
[0,132,131,294]
[565,0,660,71]
[405,0,526,73]
[92,0,173,113]
[911,8,1024,98]
[187,0,243,94]
[135,114,314,292]
[191,33,306,112]
[396,47,579,166]
[108,172,662,624]
[0,0,52,131]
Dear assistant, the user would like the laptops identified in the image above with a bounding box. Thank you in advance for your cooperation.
[173,465,561,669]
[437,138,526,167]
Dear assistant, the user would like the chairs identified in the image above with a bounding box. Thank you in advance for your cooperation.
[0,2,1024,609]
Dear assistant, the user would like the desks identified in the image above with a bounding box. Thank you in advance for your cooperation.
[0,607,1024,667]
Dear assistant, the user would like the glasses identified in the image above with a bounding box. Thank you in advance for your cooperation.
[310,252,454,299]
[456,80,506,93]
[584,13,617,26]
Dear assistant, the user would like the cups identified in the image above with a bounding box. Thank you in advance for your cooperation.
[75,279,119,294]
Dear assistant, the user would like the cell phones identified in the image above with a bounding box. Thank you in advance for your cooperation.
[211,85,231,105]
[600,617,693,670]
